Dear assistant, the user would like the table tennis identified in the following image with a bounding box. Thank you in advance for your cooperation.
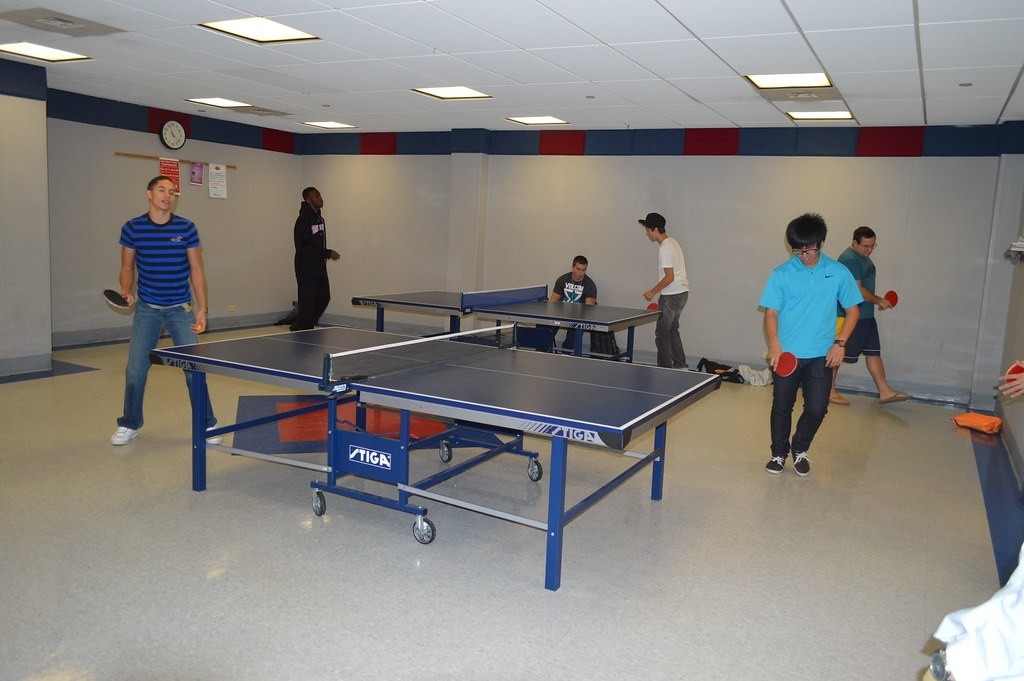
[196,324,202,330]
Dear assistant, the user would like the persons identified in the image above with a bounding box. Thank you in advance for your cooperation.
[757,213,863,476]
[998,359,1024,398]
[547,255,597,353]
[638,213,688,368]
[289,187,341,331]
[828,227,912,406]
[111,176,222,445]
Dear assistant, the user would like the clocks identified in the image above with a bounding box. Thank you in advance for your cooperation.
[159,118,186,150]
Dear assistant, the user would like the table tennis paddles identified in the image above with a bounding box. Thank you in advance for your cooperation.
[647,303,659,311]
[773,351,798,377]
[1005,361,1024,395]
[102,289,131,308]
[878,290,899,311]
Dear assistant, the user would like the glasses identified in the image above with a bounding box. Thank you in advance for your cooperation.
[859,243,877,250]
[792,247,819,255]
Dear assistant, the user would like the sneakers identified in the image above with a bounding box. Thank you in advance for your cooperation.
[111,426,141,445]
[766,455,785,473]
[205,426,222,444]
[790,444,811,477]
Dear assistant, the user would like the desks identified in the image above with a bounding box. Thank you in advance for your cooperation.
[352,284,663,365]
[150,321,719,592]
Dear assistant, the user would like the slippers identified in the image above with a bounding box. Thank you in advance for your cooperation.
[879,393,912,403]
[829,396,850,406]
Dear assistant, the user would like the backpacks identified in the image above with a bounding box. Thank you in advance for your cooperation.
[590,330,621,361]
[950,412,1003,436]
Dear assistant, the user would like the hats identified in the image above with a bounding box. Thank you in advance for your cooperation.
[638,213,666,229]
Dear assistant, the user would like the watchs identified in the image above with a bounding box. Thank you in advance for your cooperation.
[833,340,847,347]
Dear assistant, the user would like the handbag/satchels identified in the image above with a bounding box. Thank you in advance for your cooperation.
[697,358,744,384]
